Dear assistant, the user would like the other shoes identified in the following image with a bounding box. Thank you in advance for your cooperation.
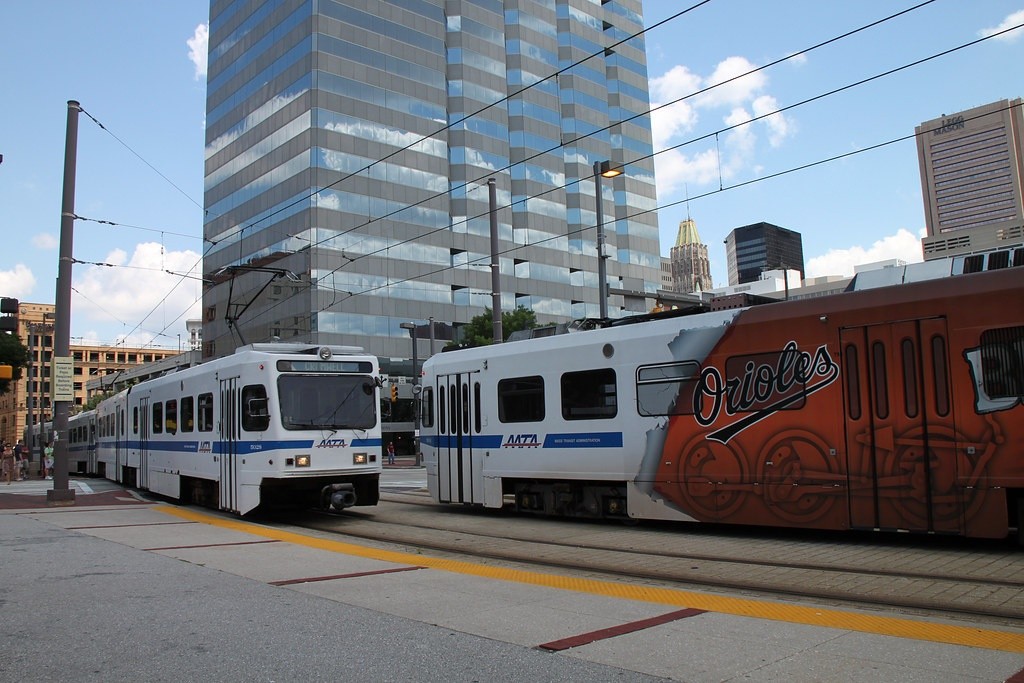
[24,476,28,478]
[45,476,53,480]
[392,463,395,464]
[17,478,23,481]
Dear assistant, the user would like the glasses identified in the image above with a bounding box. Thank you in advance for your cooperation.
[7,446,11,447]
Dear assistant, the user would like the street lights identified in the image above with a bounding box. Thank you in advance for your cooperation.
[40,311,56,471]
[400,323,421,466]
[592,159,625,319]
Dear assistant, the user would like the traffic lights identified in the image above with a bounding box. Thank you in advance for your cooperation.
[391,385,399,404]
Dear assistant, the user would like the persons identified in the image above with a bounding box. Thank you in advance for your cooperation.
[0,438,53,485]
[387,441,395,465]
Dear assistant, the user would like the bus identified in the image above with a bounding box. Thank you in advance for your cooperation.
[420,239,1024,540]
[22,342,383,519]
